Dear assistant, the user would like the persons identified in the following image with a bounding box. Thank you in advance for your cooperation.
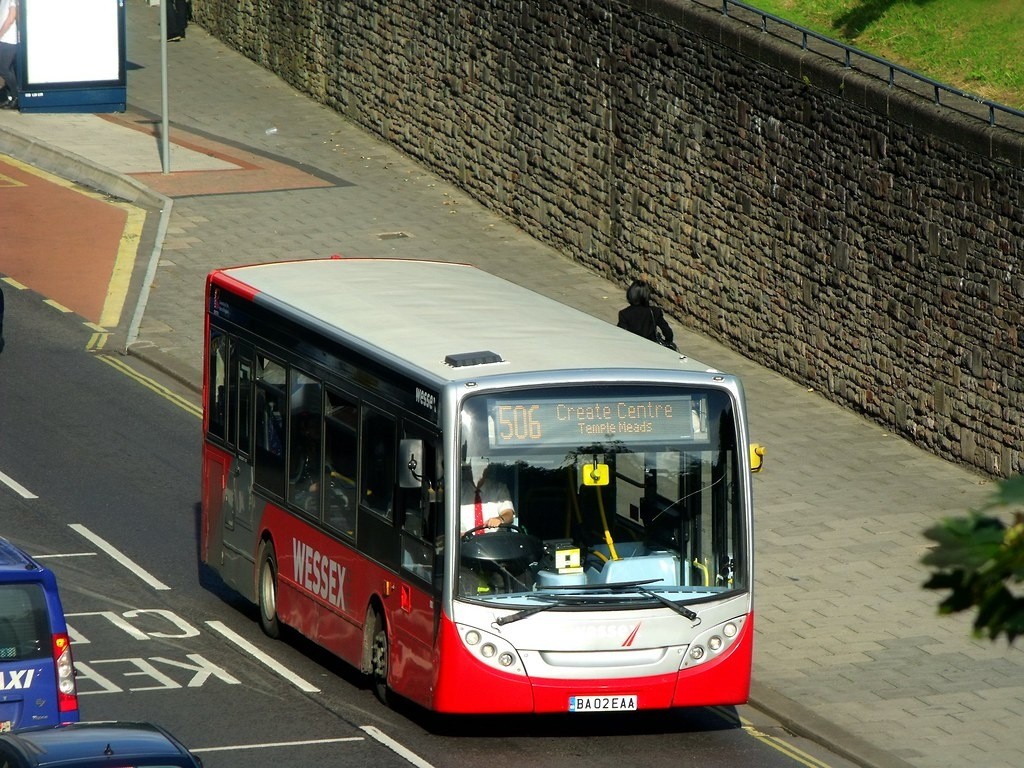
[1,0,22,108]
[166,0,195,43]
[456,457,518,555]
[616,281,673,348]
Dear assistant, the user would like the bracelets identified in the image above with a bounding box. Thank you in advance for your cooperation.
[498,513,507,525]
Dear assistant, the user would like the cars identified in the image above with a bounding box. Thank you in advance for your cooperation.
[0,722,207,768]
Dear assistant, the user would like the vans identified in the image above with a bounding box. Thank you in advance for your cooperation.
[1,536,81,735]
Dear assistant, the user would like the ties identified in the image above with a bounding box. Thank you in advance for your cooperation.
[474,489,484,535]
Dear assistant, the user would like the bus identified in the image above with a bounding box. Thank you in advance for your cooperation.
[198,261,766,721]
